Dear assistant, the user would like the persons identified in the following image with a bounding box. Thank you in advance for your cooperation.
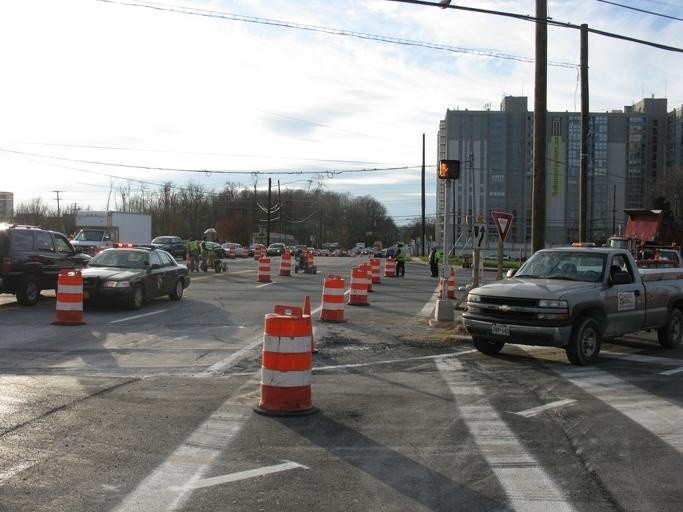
[198,236,213,272]
[427,247,441,278]
[185,238,200,273]
[393,243,407,277]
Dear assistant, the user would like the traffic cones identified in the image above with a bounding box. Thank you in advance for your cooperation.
[299,294,321,356]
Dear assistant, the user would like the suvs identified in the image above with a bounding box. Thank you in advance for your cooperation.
[0,219,97,305]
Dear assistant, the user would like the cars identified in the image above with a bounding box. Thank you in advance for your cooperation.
[149,233,419,274]
[52,242,191,312]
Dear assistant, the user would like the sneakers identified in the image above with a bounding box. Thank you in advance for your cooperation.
[392,272,441,278]
[190,267,209,273]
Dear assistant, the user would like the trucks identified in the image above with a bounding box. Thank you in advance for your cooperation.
[463,245,683,367]
[565,206,683,269]
[67,209,155,254]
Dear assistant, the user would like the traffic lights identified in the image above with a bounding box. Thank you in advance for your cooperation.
[437,159,460,178]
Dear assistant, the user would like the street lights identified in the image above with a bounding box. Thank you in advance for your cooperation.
[670,190,679,222]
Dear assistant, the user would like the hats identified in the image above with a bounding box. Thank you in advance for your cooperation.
[397,240,403,245]
